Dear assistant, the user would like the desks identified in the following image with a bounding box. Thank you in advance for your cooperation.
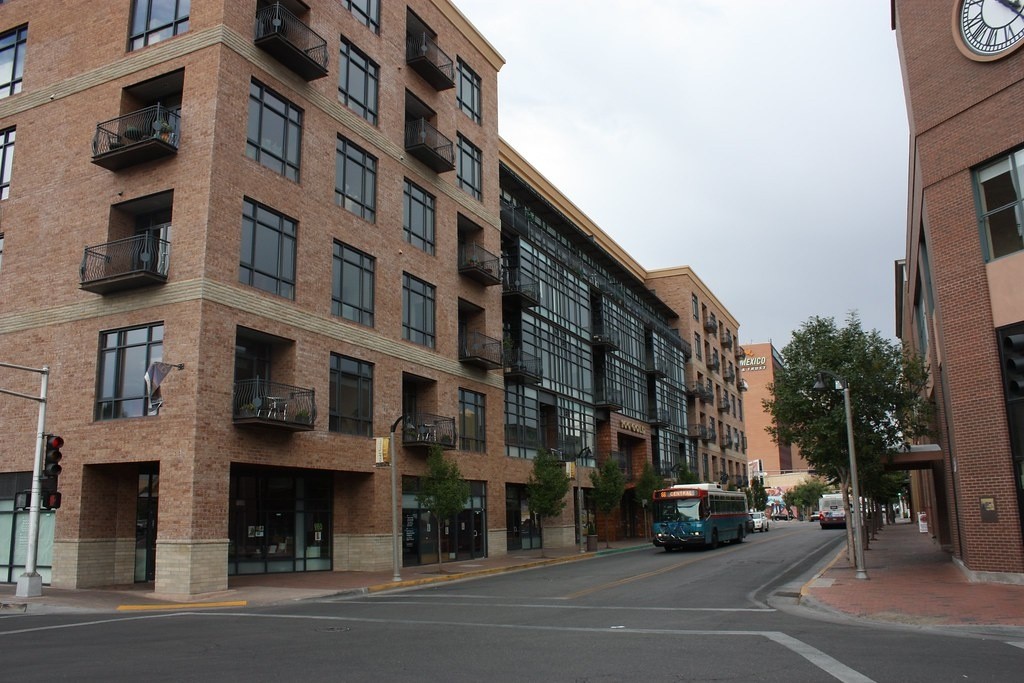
[267,396,286,419]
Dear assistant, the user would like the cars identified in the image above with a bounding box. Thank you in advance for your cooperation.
[748,512,770,533]
[810,510,819,522]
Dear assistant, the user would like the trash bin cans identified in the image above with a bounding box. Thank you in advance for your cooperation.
[587,535,598,552]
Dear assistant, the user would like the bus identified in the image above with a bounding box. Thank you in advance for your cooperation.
[817,493,855,528]
[641,483,749,553]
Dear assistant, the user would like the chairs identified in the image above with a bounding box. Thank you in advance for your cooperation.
[257,402,288,421]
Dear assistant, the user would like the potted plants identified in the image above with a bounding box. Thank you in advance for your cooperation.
[295,410,309,423]
[441,434,452,443]
[239,402,256,417]
[403,431,417,441]
[587,522,599,552]
[470,258,480,267]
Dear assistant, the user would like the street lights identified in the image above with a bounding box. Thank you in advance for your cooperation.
[575,444,592,555]
[812,368,870,580]
[389,412,416,582]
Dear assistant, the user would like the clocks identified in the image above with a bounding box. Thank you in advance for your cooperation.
[951,0,1024,62]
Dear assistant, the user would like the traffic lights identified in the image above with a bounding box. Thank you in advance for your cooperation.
[41,492,62,508]
[44,433,64,479]
[14,492,30,508]
